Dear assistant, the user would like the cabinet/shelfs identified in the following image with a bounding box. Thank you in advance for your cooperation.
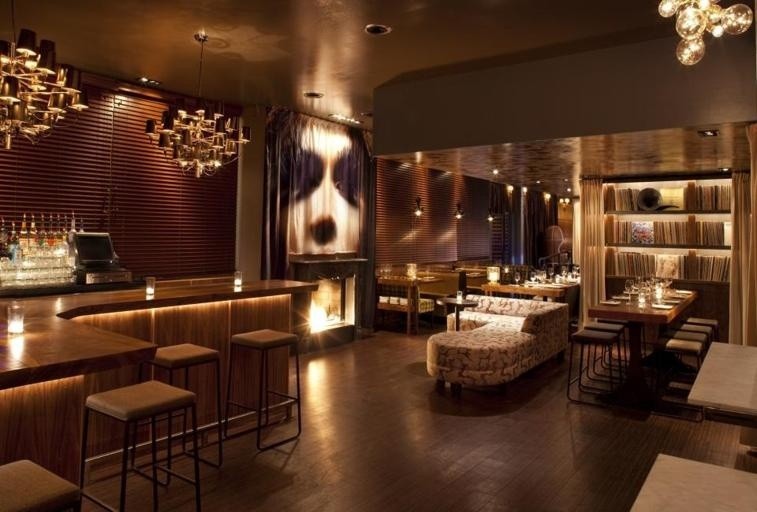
[591,169,732,289]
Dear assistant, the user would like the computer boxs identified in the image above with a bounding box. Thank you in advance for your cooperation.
[76,266,132,284]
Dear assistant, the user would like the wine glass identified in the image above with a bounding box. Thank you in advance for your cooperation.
[624,273,671,305]
[513,264,581,287]
[375,261,392,280]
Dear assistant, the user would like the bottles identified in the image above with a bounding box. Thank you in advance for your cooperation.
[1,211,85,285]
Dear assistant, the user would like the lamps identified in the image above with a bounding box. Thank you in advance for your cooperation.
[656,0,756,66]
[414,195,424,216]
[138,28,259,185]
[485,207,495,221]
[234,267,242,287]
[143,276,156,297]
[0,1,94,155]
[454,201,463,220]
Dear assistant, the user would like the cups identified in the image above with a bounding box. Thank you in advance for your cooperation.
[144,278,154,293]
[232,271,244,285]
[455,291,462,303]
[6,305,23,333]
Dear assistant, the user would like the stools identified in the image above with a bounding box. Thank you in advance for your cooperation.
[566,318,630,411]
[0,458,83,512]
[75,376,206,511]
[138,343,229,490]
[648,317,719,423]
[221,327,309,458]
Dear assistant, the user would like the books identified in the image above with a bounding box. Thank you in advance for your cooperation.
[604,181,732,283]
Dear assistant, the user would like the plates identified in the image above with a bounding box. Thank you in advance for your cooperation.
[637,188,663,213]
[597,288,691,310]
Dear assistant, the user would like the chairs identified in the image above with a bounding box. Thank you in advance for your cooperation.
[426,294,570,399]
[373,260,581,337]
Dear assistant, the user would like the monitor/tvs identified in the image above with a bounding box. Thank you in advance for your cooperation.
[71,232,120,265]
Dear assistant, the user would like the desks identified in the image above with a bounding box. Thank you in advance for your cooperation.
[686,341,756,429]
[586,289,698,422]
[627,449,757,512]
